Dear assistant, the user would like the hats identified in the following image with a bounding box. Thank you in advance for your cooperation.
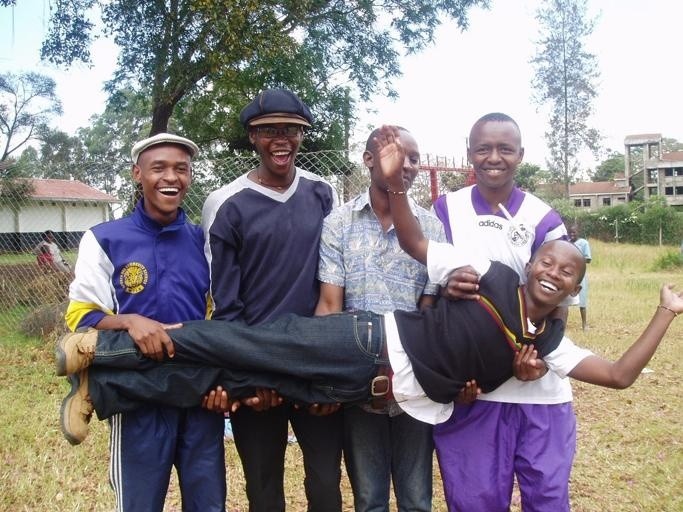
[241,87,313,133]
[128,132,200,164]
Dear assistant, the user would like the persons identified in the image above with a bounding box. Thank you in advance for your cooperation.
[63,129,227,511]
[199,86,344,511]
[566,223,592,332]
[36,244,55,273]
[424,110,580,512]
[52,122,682,446]
[30,229,70,274]
[307,122,448,511]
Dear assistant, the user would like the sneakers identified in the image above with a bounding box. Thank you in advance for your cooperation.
[59,372,95,446]
[55,330,98,377]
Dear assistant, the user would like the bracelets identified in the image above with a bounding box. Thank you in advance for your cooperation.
[386,188,405,197]
[657,304,678,320]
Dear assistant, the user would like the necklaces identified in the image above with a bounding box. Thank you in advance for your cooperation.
[253,167,296,190]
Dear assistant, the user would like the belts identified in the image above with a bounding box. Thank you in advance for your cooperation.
[371,343,393,409]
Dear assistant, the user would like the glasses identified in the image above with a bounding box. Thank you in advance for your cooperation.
[253,123,302,138]
